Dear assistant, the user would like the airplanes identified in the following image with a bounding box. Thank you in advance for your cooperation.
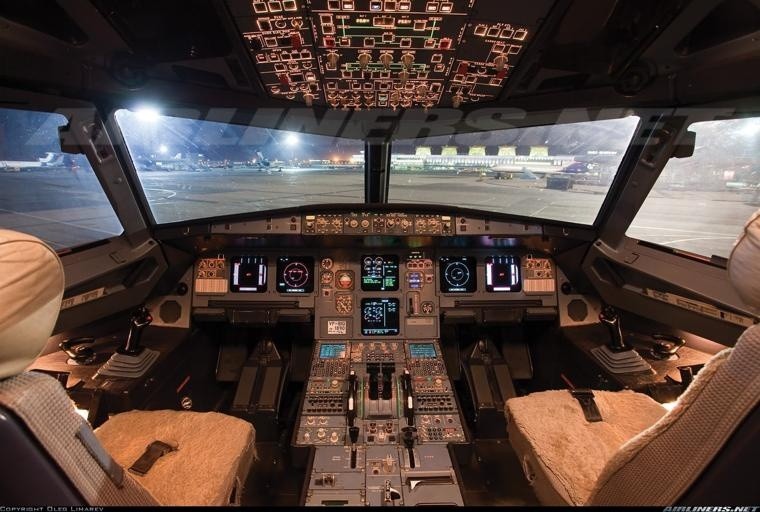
[0,151,71,172]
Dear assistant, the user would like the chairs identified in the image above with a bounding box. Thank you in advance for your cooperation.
[0,228,261,509]
[503,208,760,507]
